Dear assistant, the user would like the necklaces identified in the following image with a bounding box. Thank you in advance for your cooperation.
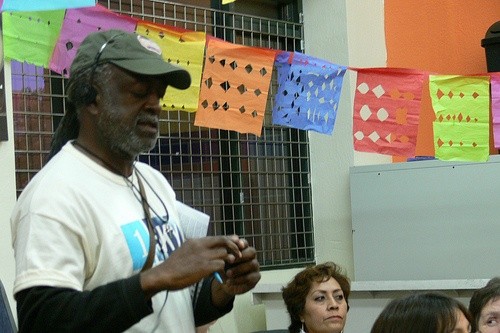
[74,136,171,223]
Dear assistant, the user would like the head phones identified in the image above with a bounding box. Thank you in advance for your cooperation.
[81,31,124,105]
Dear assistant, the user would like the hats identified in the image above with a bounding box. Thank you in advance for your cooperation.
[68,29,191,91]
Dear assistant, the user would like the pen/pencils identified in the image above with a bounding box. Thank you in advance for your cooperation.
[213,272,223,285]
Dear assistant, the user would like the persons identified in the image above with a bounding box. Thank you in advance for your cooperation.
[468,275,500,333]
[11,28,261,333]
[279,262,351,333]
[370,290,476,333]
